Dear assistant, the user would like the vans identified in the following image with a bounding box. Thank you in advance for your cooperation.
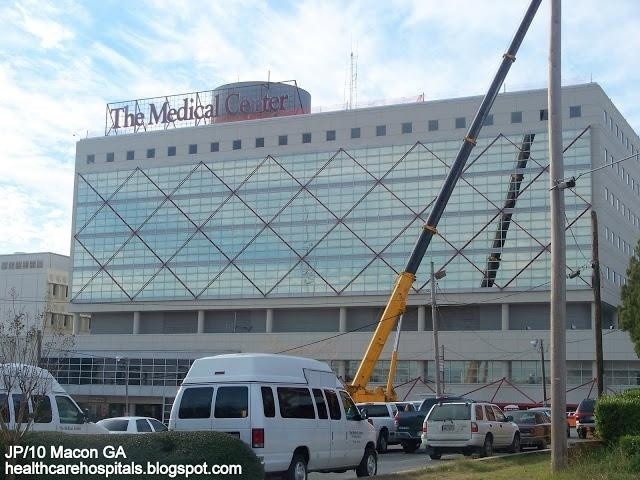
[1,363,110,435]
[167,354,379,480]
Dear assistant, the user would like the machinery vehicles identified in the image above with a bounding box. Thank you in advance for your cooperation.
[463,136,535,384]
[342,0,542,404]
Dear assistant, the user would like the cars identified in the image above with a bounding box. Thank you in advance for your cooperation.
[354,397,598,460]
[95,416,168,434]
